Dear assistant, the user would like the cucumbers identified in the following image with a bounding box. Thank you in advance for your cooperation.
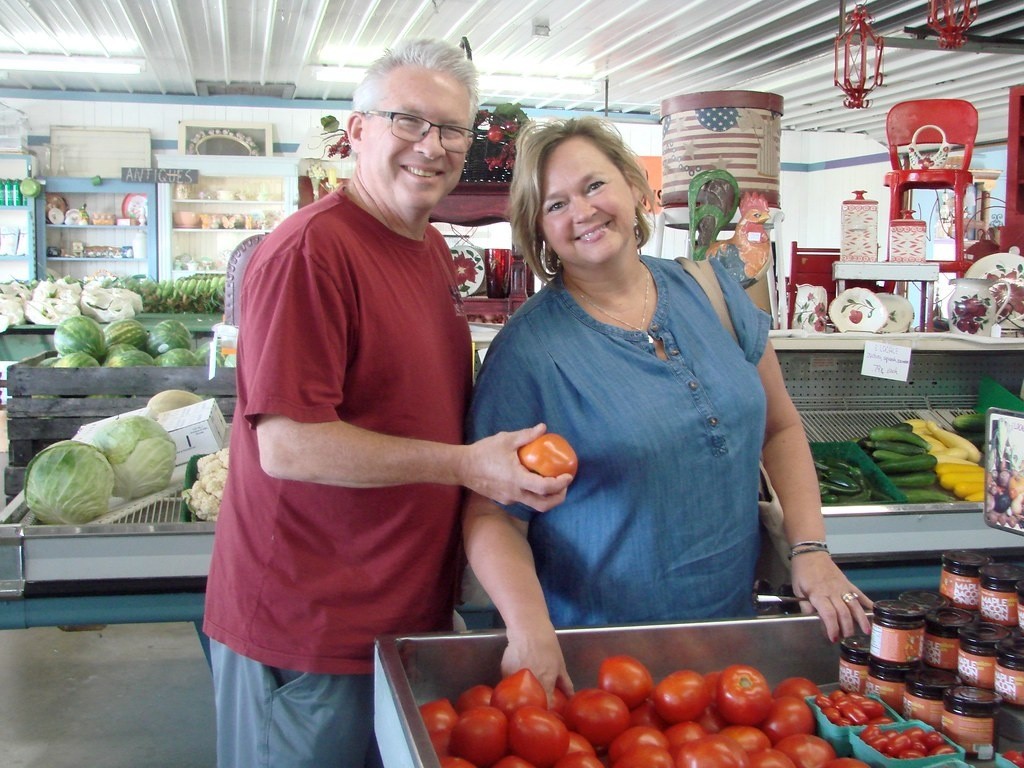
[851,423,956,502]
[951,413,986,453]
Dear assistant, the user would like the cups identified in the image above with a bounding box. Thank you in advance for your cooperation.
[484,249,510,298]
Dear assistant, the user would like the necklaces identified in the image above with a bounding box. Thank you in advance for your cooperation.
[575,268,654,344]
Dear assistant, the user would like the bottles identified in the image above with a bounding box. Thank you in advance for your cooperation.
[889,210,926,263]
[840,190,879,263]
[0,178,27,206]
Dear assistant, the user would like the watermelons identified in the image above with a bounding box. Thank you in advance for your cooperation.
[31,316,237,399]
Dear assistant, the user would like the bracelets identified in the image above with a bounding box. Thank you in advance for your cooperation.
[788,540,830,559]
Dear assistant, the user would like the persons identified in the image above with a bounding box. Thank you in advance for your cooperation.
[462,118,874,711]
[202,37,574,766]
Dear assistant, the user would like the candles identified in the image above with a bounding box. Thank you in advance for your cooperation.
[328,169,337,185]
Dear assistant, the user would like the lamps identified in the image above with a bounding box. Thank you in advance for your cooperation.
[296,135,340,202]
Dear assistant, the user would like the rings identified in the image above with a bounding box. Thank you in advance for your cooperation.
[842,592,859,603]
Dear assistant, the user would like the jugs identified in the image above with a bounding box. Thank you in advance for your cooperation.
[947,278,1011,337]
[791,282,827,334]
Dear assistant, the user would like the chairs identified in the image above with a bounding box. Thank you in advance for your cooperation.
[883,99,974,332]
[777,241,896,329]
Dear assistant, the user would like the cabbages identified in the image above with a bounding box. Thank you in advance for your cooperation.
[24,416,176,525]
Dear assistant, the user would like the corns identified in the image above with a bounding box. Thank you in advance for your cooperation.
[102,276,225,313]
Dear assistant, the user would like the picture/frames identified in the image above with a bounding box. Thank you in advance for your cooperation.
[177,119,273,155]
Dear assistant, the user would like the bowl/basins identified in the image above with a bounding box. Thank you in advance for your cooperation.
[172,190,279,229]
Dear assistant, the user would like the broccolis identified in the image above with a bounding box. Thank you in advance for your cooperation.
[180,448,229,521]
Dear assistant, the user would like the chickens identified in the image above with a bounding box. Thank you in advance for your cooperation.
[687,168,775,290]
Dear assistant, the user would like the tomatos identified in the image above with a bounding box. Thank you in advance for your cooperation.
[418,655,955,768]
[517,433,578,477]
[1002,750,1024,768]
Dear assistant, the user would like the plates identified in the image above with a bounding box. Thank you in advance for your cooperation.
[122,193,147,225]
[828,287,888,333]
[964,253,1024,332]
[449,246,485,298]
[46,192,68,224]
[876,293,914,334]
[48,208,64,224]
[66,209,82,224]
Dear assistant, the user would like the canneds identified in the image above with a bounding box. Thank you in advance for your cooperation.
[838,550,1024,760]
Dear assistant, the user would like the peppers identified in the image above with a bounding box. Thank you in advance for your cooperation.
[812,455,894,503]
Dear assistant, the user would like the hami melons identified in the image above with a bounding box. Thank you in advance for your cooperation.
[146,390,203,421]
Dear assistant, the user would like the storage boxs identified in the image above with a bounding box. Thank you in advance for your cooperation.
[156,398,227,466]
[804,694,1021,768]
[659,90,783,205]
[116,219,137,226]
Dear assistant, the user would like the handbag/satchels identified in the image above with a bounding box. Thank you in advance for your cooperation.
[754,460,793,589]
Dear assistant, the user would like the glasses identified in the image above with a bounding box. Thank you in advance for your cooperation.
[361,111,478,153]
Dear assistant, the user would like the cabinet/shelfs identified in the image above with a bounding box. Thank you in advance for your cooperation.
[35,177,158,284]
[297,176,536,324]
[0,154,36,292]
[155,154,301,284]
